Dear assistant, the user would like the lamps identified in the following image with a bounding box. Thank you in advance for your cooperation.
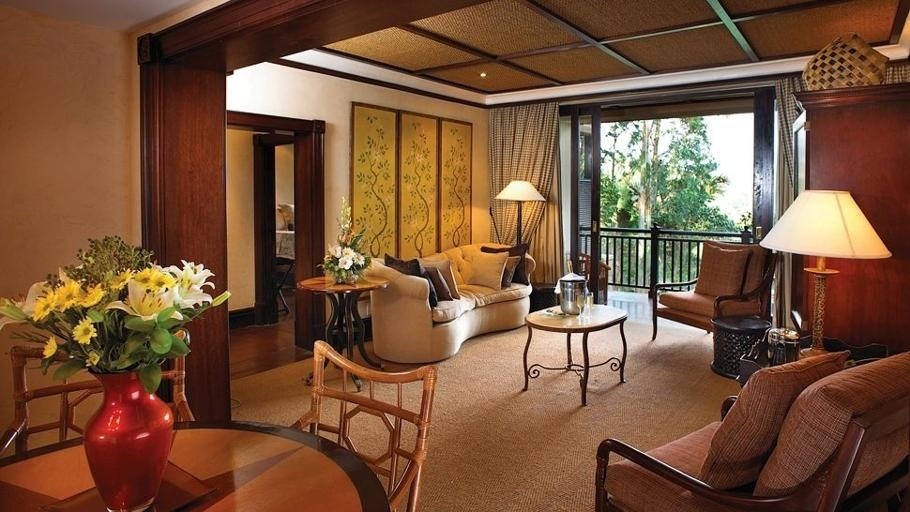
[759,190,892,359]
[494,180,546,246]
[801,32,889,91]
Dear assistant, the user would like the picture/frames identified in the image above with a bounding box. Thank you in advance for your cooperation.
[350,102,398,261]
[398,110,440,261]
[438,117,473,251]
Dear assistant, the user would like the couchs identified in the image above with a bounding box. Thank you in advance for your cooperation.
[596,349,910,512]
[364,243,537,363]
[651,240,778,342]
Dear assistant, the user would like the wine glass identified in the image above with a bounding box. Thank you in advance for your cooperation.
[576,292,586,317]
[586,292,594,317]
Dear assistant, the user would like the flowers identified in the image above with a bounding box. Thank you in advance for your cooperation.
[1,235,232,394]
[316,196,372,284]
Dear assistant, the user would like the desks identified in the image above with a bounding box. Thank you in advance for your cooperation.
[0,420,391,512]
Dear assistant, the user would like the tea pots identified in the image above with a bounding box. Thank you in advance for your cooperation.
[767,327,802,366]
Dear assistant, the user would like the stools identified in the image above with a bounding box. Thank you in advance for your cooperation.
[709,316,772,380]
[529,283,560,313]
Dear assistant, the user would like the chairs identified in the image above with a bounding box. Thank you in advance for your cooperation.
[579,253,611,304]
[0,328,195,467]
[288,340,437,512]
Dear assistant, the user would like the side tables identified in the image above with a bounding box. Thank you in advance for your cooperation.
[296,275,391,392]
[740,329,890,390]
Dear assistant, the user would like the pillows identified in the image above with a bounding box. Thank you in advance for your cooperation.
[480,244,529,286]
[501,256,521,287]
[695,243,751,299]
[384,252,438,307]
[424,266,453,302]
[466,250,509,290]
[695,351,851,492]
[414,256,460,300]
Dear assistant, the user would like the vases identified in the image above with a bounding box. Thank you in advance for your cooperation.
[82,369,173,512]
